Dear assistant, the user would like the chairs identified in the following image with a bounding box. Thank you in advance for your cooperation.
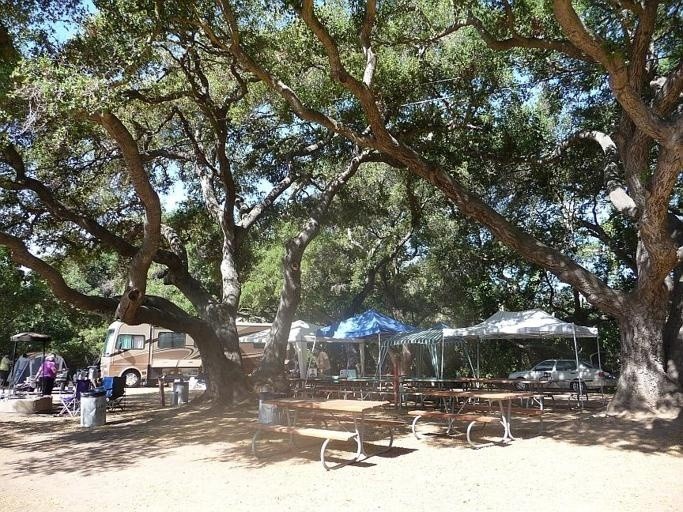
[57,379,90,418]
[107,376,129,413]
[93,376,114,405]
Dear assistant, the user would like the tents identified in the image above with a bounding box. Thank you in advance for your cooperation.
[6,349,67,386]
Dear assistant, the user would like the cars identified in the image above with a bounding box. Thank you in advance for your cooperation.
[507,357,614,392]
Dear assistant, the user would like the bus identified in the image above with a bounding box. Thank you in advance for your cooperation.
[100,316,296,389]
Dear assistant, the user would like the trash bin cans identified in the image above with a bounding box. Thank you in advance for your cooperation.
[76,380,90,401]
[173,382,189,406]
[39,377,52,395]
[104,377,125,403]
[257,392,286,424]
[80,391,106,427]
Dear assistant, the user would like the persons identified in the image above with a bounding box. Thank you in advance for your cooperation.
[33,354,58,395]
[314,345,332,382]
[0,353,12,386]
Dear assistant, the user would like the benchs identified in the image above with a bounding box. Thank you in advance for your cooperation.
[394,377,594,448]
[246,377,394,472]
